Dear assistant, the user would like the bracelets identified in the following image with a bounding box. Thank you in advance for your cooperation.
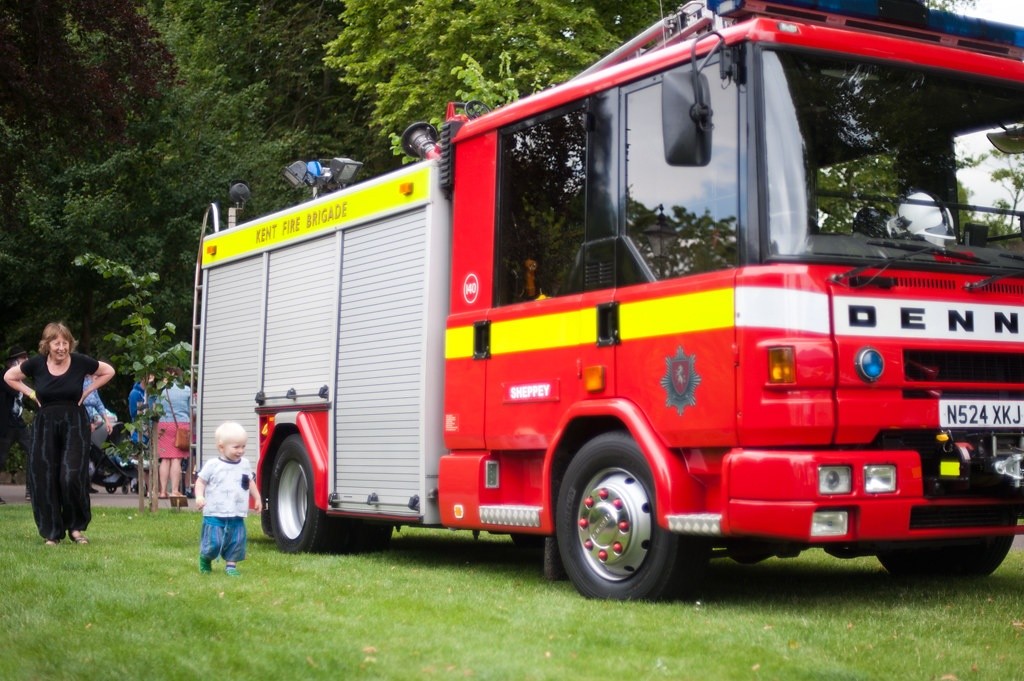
[195,497,204,501]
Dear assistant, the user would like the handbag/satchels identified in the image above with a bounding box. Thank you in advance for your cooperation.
[174,429,190,449]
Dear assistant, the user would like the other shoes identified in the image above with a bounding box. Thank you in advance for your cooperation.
[158,492,171,498]
[200,556,212,575]
[89,487,98,493]
[68,531,90,544]
[0,498,5,504]
[171,493,188,497]
[25,496,31,500]
[44,538,60,545]
[226,568,240,577]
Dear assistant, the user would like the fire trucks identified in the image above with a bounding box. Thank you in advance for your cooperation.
[185,0,1024,603]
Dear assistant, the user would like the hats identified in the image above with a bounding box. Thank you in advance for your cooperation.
[9,346,26,357]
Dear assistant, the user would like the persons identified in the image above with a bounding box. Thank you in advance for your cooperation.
[83,374,113,493]
[0,346,33,504]
[128,367,197,499]
[4,323,115,544]
[194,423,262,575]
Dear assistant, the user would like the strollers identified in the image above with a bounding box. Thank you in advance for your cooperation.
[89,421,141,494]
